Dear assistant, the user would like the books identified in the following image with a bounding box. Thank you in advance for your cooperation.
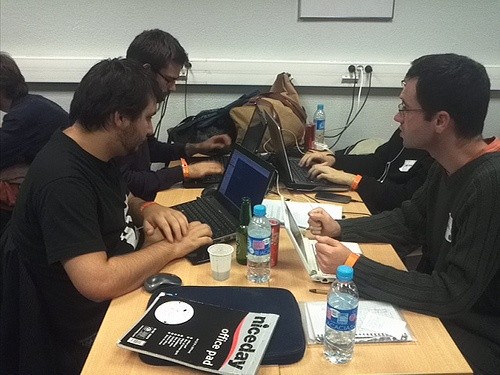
[116,292,280,375]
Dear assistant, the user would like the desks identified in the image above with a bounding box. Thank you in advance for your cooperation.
[79,155,475,375]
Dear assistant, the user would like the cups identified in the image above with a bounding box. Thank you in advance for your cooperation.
[207,243,234,280]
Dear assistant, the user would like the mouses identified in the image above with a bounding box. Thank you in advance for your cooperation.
[202,184,220,196]
[144,272,182,293]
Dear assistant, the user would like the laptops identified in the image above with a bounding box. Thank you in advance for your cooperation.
[280,195,362,283]
[158,104,349,244]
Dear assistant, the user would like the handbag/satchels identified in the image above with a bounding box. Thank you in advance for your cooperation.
[168,72,308,155]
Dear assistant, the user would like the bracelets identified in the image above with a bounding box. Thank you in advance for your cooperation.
[350,174,362,191]
[181,158,190,182]
[140,201,157,216]
[344,253,360,267]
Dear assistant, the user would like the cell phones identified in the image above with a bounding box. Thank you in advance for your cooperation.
[315,192,352,203]
[187,246,211,266]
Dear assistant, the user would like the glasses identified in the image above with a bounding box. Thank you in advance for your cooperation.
[147,63,175,86]
[397,103,432,116]
[400,80,407,87]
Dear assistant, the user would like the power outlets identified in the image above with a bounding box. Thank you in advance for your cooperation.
[341,64,360,80]
[357,64,375,74]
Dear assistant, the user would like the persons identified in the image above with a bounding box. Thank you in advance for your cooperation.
[0,58,214,375]
[298,55,437,217]
[0,54,72,171]
[112,29,231,202]
[307,53,500,375]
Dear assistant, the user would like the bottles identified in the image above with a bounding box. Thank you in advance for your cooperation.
[236,196,252,264]
[246,205,271,283]
[312,104,326,144]
[323,265,359,365]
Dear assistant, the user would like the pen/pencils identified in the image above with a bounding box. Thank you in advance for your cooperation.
[309,289,328,294]
